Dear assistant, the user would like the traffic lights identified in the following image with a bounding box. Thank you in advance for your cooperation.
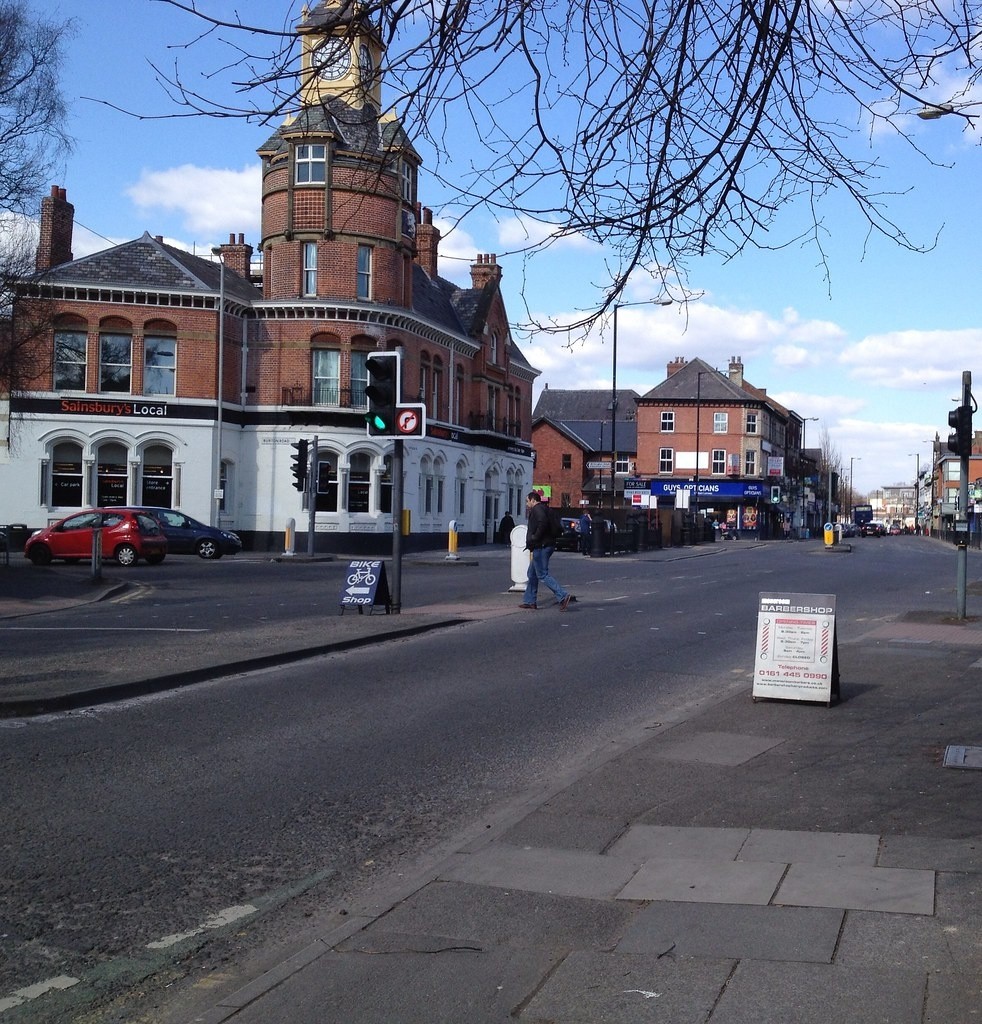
[948,405,972,458]
[770,486,780,503]
[290,439,308,492]
[363,351,398,436]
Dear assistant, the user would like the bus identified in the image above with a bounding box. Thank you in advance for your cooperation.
[855,503,874,528]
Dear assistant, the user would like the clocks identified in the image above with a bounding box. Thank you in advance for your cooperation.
[360,45,373,87]
[311,37,351,80]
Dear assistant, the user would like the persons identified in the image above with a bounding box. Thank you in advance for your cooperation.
[499,511,515,548]
[519,492,571,612]
[713,518,719,526]
[784,518,791,539]
[580,509,592,555]
[916,524,920,536]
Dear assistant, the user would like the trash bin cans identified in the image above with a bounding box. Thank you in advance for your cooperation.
[10,524,28,552]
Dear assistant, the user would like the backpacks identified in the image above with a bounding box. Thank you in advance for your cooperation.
[541,505,565,540]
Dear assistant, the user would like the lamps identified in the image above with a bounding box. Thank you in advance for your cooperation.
[245,385,256,398]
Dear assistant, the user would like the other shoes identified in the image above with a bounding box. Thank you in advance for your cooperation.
[583,553,587,555]
[560,594,572,612]
[518,604,538,610]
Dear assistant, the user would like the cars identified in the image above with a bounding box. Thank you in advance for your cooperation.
[554,517,618,553]
[865,524,901,538]
[24,505,244,566]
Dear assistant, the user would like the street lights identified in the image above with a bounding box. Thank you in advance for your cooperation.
[850,456,860,524]
[908,452,920,534]
[693,368,742,545]
[610,298,674,518]
[801,416,820,530]
[211,246,229,531]
